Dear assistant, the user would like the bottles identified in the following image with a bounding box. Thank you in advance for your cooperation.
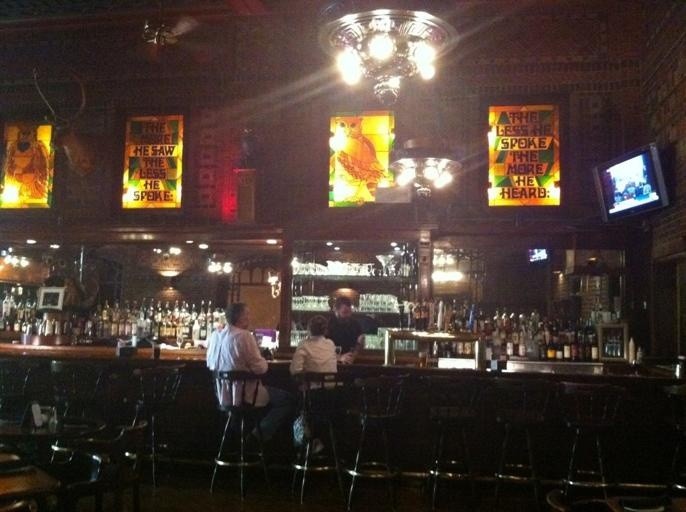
[388,327,417,351]
[401,242,417,277]
[411,299,644,366]
[132,334,138,348]
[1,282,223,338]
[401,283,417,311]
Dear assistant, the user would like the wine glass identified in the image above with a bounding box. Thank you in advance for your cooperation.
[291,330,310,348]
[365,327,386,349]
[176,333,184,353]
[293,290,399,315]
[294,254,398,275]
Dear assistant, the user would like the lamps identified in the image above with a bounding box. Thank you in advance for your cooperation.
[32,67,106,178]
[317,0,460,107]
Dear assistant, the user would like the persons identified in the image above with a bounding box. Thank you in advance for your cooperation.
[289,315,337,457]
[324,296,365,365]
[205,302,296,443]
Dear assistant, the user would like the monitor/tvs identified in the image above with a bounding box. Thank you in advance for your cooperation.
[527,248,551,264]
[592,142,669,224]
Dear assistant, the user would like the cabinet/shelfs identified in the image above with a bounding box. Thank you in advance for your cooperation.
[288,240,418,353]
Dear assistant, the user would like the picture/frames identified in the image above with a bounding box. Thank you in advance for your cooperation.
[37,286,66,311]
